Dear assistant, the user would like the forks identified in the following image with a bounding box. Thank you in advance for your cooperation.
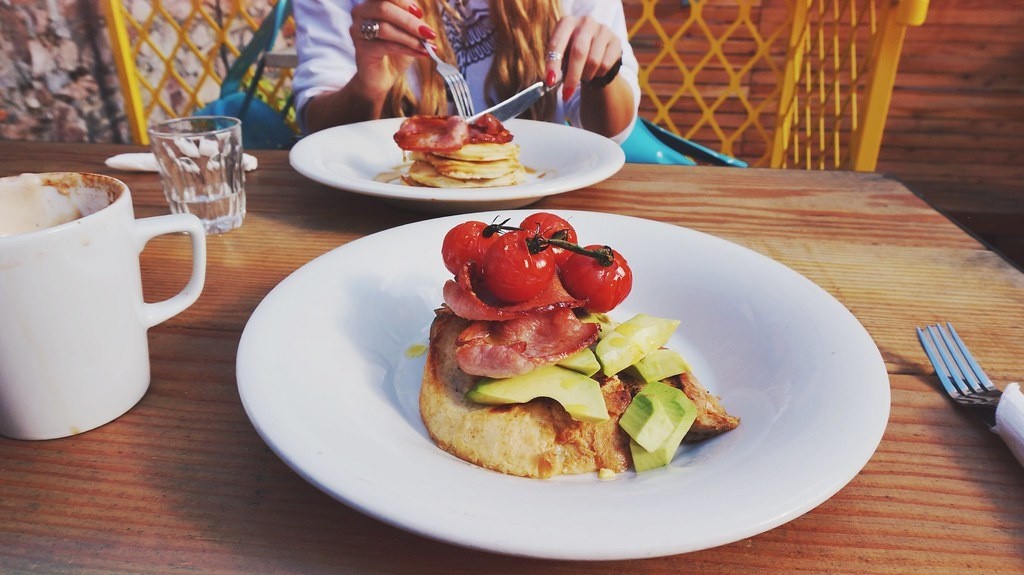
[918,325,1023,461]
[395,40,475,119]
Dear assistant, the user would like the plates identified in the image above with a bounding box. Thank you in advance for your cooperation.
[237,210,891,561]
[288,119,625,208]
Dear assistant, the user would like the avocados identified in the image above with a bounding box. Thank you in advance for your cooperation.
[467,313,699,474]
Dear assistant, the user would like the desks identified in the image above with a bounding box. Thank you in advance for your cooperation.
[0,139,1024,575]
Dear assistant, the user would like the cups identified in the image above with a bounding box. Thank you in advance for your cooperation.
[150,116,244,234]
[1,171,207,440]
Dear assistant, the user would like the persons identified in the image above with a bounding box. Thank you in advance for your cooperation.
[290,0,641,146]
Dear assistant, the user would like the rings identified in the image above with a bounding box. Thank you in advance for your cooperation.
[545,51,562,62]
[361,20,379,40]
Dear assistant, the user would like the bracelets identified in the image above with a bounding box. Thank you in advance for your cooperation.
[580,57,622,87]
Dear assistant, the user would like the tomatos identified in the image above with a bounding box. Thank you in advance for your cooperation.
[441,212,632,313]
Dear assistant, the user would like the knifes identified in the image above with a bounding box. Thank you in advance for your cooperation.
[467,69,567,126]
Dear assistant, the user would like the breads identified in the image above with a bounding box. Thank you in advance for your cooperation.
[421,355,634,480]
[404,142,526,189]
